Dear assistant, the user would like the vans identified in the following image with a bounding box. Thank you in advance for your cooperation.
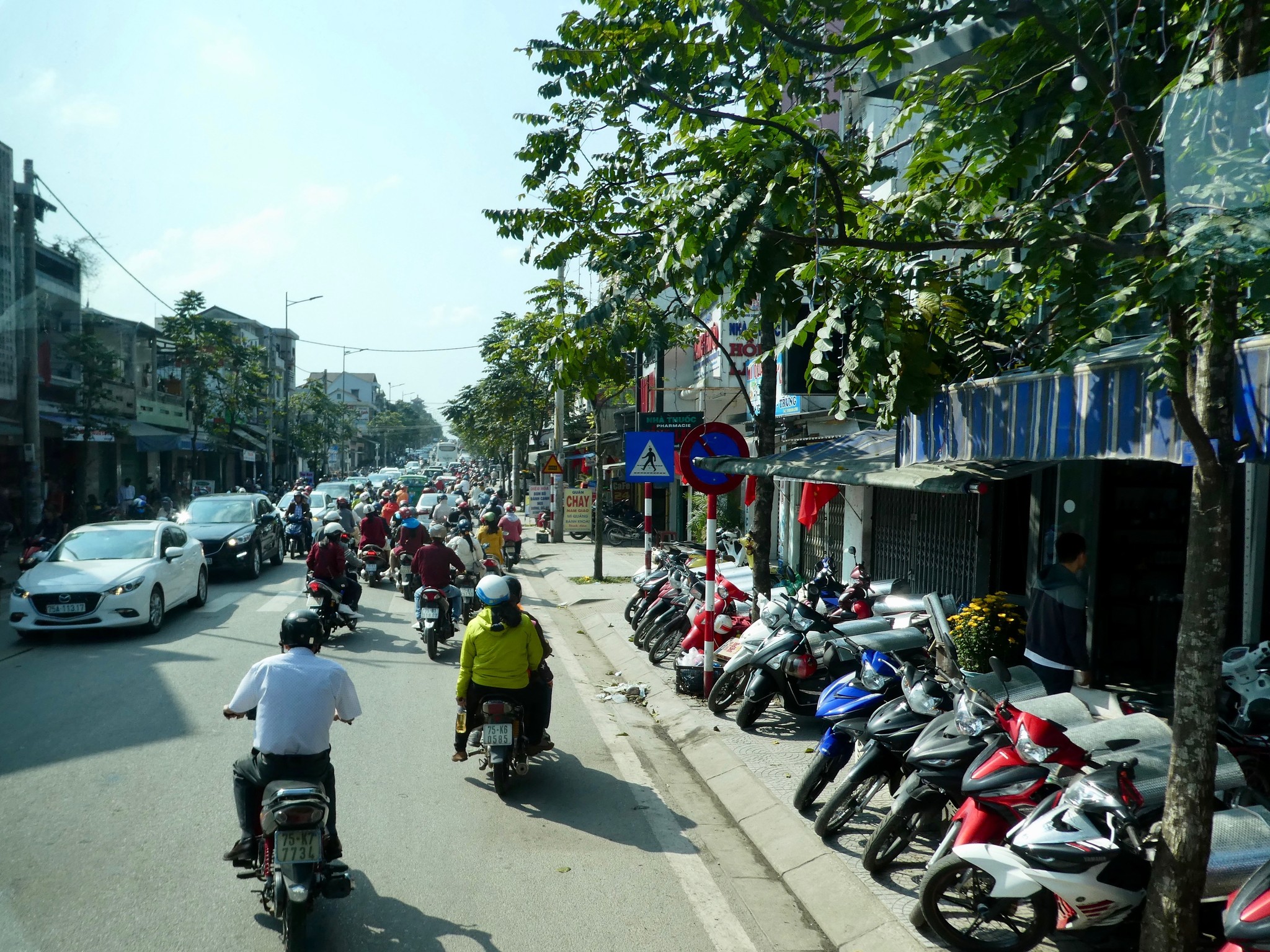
[398,475,428,505]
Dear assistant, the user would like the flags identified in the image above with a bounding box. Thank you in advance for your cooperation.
[798,482,840,533]
[744,474,757,507]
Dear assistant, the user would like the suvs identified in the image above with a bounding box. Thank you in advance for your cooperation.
[342,477,369,485]
[504,471,536,495]
[366,467,399,491]
[312,481,356,508]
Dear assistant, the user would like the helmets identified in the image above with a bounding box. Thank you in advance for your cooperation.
[851,600,871,619]
[132,495,146,507]
[717,540,727,552]
[244,458,515,539]
[475,575,510,605]
[794,653,817,679]
[501,575,523,604]
[714,614,732,635]
[279,610,324,650]
[161,496,171,503]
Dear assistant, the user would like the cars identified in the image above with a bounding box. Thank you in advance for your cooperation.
[271,491,336,547]
[8,493,285,639]
[386,444,473,531]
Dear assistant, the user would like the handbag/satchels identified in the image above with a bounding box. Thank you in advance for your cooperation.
[473,561,483,574]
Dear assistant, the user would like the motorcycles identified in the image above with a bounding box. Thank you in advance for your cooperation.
[78,494,121,524]
[413,568,463,658]
[302,552,365,643]
[467,647,554,794]
[625,540,1270,952]
[253,462,522,625]
[224,706,352,952]
[569,496,665,547]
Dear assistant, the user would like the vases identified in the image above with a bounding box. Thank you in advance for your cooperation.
[960,669,1005,696]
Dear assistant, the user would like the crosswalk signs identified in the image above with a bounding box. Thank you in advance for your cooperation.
[624,432,674,482]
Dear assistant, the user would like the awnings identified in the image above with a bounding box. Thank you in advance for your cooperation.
[233,424,286,449]
[693,429,1060,493]
[39,413,217,452]
[528,436,620,466]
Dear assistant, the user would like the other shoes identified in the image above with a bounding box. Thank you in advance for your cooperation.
[527,738,555,757]
[380,571,396,577]
[466,732,483,747]
[452,622,460,632]
[412,622,421,629]
[452,751,468,762]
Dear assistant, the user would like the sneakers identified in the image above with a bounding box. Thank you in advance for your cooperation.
[338,603,355,615]
[347,611,364,618]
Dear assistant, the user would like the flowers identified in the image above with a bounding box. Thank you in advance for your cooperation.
[946,590,1030,672]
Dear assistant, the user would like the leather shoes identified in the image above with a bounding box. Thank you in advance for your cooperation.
[322,834,342,861]
[223,838,258,861]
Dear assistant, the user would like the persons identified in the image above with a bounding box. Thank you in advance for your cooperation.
[1024,532,1090,696]
[29,503,68,542]
[44,474,64,516]
[408,454,424,461]
[452,574,554,761]
[306,460,523,631]
[222,608,363,860]
[226,473,340,552]
[85,477,177,523]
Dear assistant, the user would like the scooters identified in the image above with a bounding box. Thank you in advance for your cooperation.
[19,537,57,575]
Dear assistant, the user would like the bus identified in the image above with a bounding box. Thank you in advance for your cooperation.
[436,442,457,462]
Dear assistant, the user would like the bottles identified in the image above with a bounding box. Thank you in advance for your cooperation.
[681,661,722,674]
[456,699,467,733]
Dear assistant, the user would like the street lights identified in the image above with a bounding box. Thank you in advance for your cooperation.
[341,346,369,478]
[388,382,414,409]
[284,292,323,481]
